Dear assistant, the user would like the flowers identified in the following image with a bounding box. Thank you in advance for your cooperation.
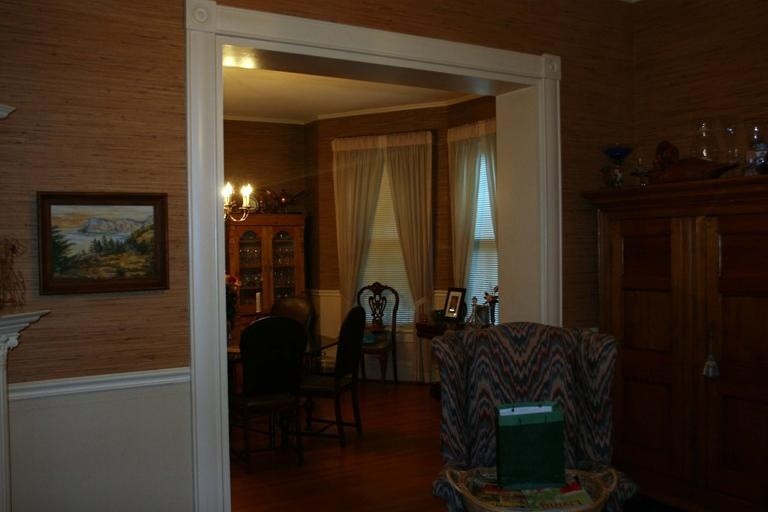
[482,286,500,305]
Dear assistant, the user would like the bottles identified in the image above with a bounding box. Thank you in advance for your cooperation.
[279,188,291,214]
[467,304,482,329]
[692,119,763,161]
[240,247,294,300]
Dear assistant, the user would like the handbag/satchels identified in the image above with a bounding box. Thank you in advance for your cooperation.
[494,402,565,491]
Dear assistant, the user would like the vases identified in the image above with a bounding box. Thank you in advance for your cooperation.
[490,303,495,326]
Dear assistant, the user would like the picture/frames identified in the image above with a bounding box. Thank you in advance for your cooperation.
[36,191,170,297]
[443,287,467,320]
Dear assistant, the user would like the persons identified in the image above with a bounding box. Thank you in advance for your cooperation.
[449,297,457,313]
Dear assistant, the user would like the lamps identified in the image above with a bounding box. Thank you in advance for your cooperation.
[223,181,252,222]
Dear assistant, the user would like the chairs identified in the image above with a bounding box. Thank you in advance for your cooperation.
[429,321,639,512]
[227,281,398,468]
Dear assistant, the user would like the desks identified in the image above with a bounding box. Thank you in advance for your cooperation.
[416,322,465,399]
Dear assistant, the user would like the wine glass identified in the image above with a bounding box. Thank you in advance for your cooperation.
[600,143,635,186]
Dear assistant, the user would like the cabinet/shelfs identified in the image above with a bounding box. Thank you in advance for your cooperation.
[226,212,306,395]
[579,174,766,512]
